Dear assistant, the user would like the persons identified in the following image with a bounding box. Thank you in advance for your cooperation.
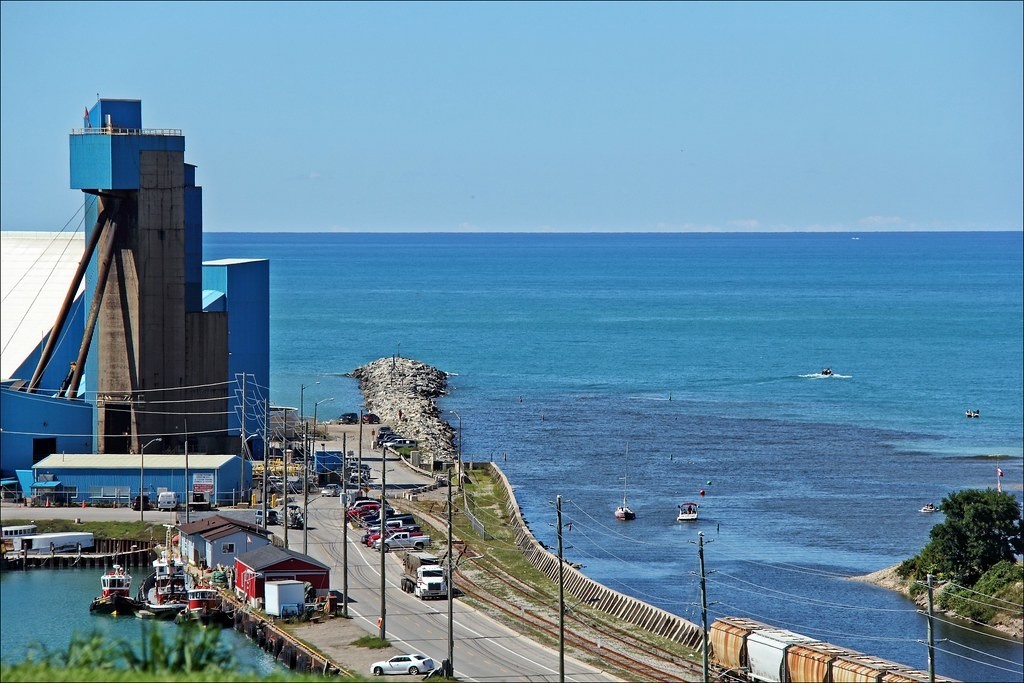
[682,505,695,514]
[926,503,938,509]
[967,408,971,413]
[974,410,979,414]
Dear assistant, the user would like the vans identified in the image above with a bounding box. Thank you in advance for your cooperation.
[338,413,358,425]
[157,491,180,511]
[254,510,278,525]
[376,427,417,448]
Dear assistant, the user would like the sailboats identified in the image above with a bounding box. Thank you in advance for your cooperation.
[615,442,635,522]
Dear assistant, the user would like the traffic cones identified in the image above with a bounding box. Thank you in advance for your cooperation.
[82,500,85,508]
[45,496,50,507]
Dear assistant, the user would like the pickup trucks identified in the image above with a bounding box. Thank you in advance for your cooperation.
[346,497,431,552]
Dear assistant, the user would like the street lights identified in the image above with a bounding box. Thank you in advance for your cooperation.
[312,398,334,456]
[301,382,320,423]
[141,438,162,522]
[450,410,461,488]
[242,434,257,501]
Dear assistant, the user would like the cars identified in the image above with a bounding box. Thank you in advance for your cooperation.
[131,495,150,511]
[348,462,372,483]
[370,654,435,676]
[362,414,380,424]
[258,475,302,492]
[321,484,340,497]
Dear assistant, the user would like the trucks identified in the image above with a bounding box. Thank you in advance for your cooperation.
[401,550,448,600]
[278,504,304,525]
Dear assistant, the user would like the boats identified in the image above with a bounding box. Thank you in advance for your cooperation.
[676,502,700,521]
[966,408,979,417]
[135,528,188,615]
[822,370,832,375]
[90,546,134,615]
[921,507,939,513]
[174,589,222,625]
